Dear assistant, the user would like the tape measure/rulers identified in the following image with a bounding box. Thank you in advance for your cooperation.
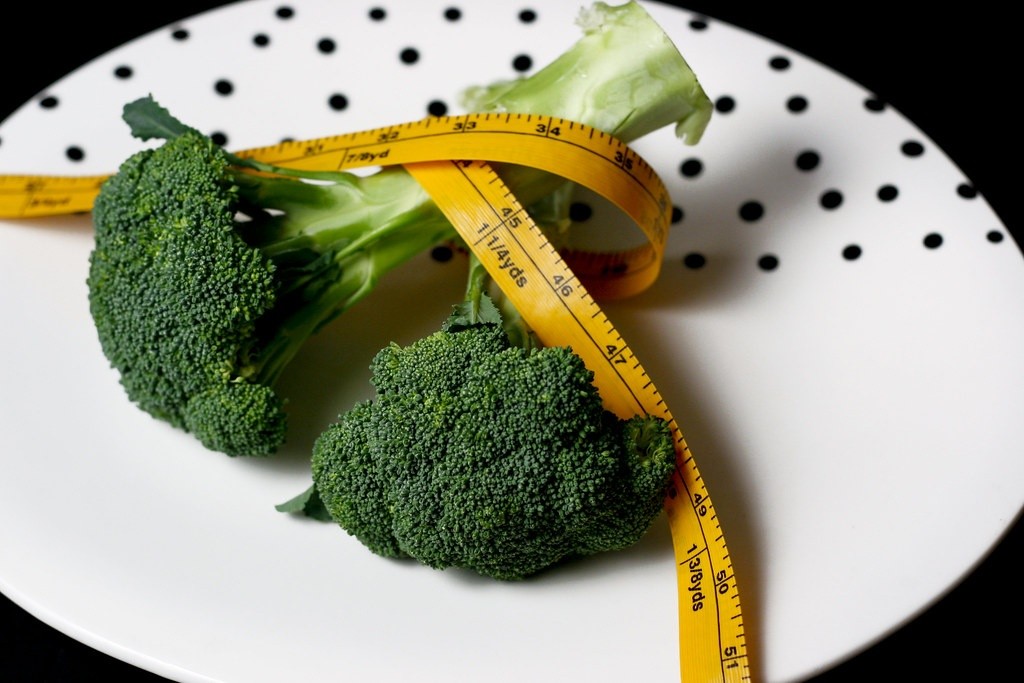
[0,109,753,683]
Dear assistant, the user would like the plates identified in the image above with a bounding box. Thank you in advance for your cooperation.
[0,0,1024,683]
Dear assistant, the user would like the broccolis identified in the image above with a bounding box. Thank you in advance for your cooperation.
[86,0,712,582]
